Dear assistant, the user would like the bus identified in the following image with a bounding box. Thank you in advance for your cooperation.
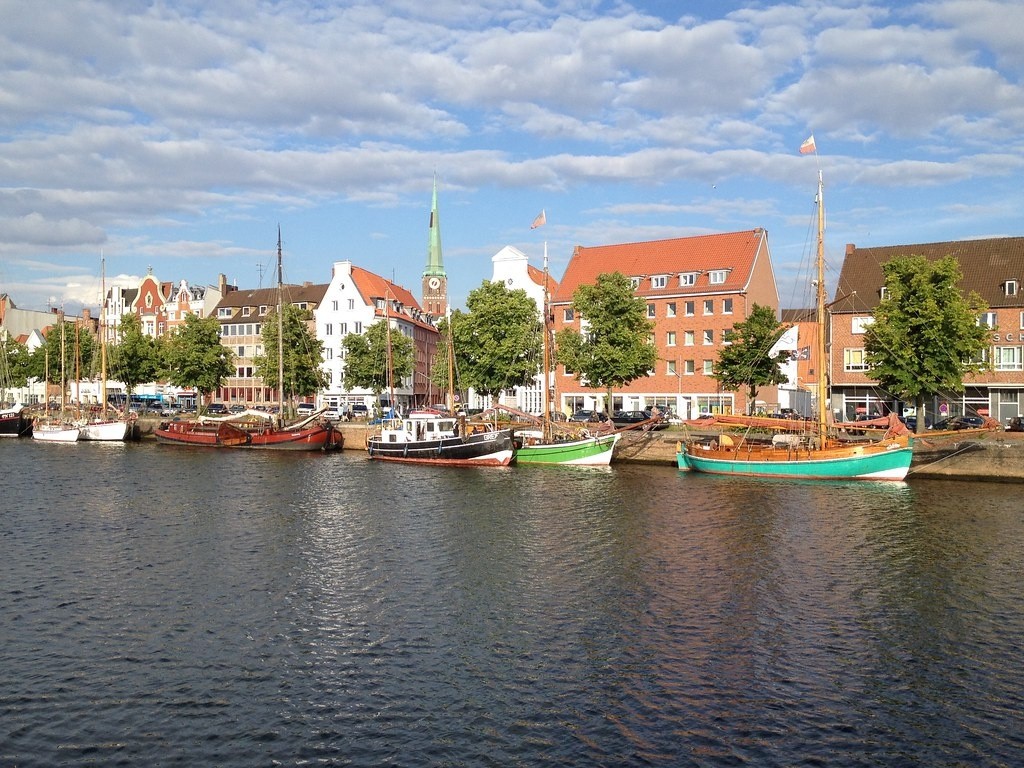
[107,393,200,410]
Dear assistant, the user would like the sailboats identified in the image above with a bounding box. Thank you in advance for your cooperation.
[366,301,514,468]
[676,170,914,481]
[32,259,129,443]
[517,240,620,465]
[154,223,343,451]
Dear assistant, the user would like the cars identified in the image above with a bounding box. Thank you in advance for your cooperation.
[126,402,487,421]
[537,406,669,435]
[856,412,985,435]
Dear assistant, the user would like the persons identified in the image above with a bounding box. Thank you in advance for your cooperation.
[453,426,459,438]
[472,427,478,435]
[337,402,352,422]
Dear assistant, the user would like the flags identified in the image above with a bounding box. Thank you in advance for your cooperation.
[531,210,545,229]
[799,134,816,153]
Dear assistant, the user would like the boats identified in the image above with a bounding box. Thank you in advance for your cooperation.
[0,401,23,438]
